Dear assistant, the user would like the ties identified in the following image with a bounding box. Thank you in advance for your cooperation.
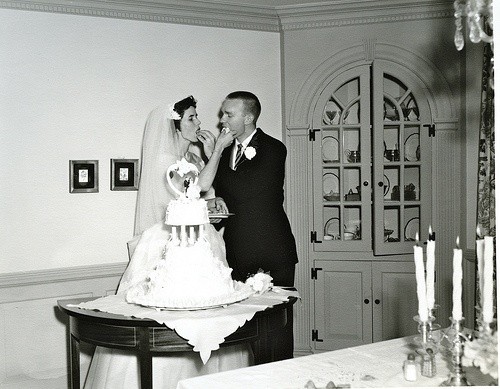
[234,144,243,170]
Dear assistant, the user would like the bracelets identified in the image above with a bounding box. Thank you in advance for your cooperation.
[212,150,222,158]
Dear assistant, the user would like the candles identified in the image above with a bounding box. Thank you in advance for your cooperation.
[412,223,495,323]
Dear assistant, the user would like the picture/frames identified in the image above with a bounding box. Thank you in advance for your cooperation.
[110,158,139,192]
[69,160,99,194]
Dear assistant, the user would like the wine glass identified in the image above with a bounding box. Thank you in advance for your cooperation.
[338,111,350,124]
[402,107,412,121]
[326,110,337,126]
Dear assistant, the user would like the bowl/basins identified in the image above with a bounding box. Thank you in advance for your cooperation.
[324,193,340,201]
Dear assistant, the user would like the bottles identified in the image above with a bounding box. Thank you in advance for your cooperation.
[404,353,416,382]
[349,151,360,163]
[385,150,398,161]
[421,349,436,378]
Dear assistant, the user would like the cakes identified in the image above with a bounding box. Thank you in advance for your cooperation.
[146,160,234,305]
[221,127,230,133]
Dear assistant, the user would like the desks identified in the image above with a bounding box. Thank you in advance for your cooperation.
[177,326,495,389]
[57,284,299,389]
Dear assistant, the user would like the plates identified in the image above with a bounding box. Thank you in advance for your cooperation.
[324,217,346,239]
[404,132,419,162]
[404,217,419,240]
[321,135,340,161]
[322,173,340,198]
[384,100,405,120]
[383,174,390,197]
[208,213,235,218]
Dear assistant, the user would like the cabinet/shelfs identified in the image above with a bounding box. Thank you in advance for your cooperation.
[308,58,433,255]
[310,257,440,353]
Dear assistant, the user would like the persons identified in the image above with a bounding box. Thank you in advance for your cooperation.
[83,95,237,389]
[196,91,299,367]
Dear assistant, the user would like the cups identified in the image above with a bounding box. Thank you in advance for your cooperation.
[324,235,333,241]
[344,232,353,241]
